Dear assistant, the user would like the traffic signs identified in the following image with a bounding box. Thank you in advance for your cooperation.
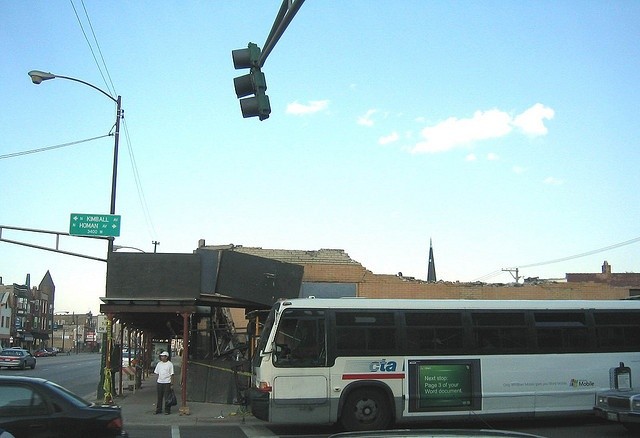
[70,213,121,236]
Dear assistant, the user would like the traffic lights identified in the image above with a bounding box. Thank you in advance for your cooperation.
[231,41,271,121]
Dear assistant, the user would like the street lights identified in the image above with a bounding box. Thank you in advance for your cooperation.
[51,310,69,347]
[28,67,123,400]
[112,243,147,253]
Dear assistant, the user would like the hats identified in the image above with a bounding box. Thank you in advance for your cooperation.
[159,351,169,357]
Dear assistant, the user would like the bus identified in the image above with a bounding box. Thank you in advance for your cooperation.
[245,297,640,433]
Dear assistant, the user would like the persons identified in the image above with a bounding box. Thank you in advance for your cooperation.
[154,350,174,414]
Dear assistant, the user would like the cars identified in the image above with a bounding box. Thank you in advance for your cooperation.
[593,387,640,435]
[36,347,57,357]
[0,374,128,437]
[0,346,37,369]
